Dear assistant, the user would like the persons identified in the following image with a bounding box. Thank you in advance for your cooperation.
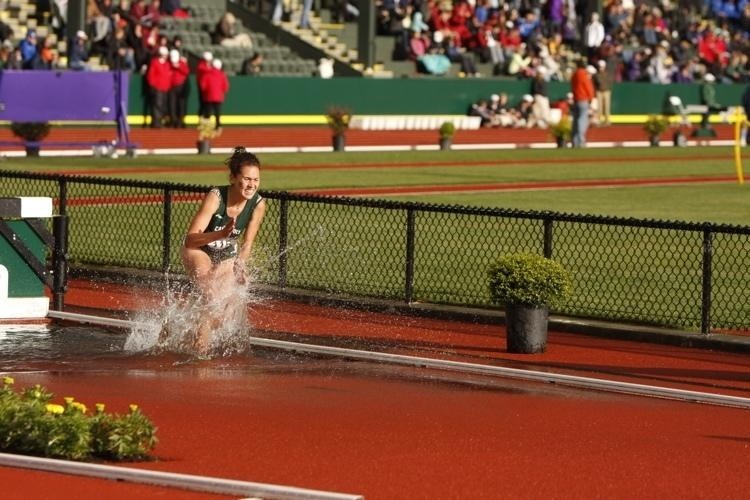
[569,57,593,149]
[178,144,266,348]
[241,52,265,76]
[376,0,750,129]
[1,0,237,130]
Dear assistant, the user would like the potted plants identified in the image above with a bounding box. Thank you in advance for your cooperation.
[486,252,572,355]
[438,117,671,155]
[10,120,51,159]
[327,106,352,150]
[197,115,222,156]
